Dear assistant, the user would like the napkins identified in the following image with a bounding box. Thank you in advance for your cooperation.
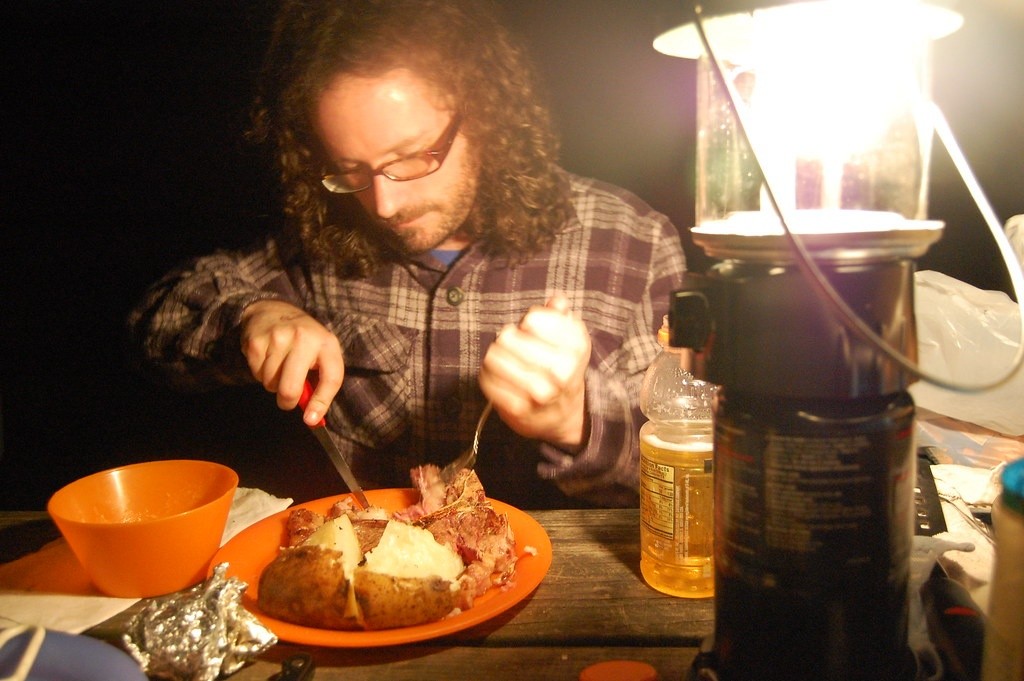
[0,486,295,634]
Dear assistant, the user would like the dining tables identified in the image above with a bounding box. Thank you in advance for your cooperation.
[0,508,716,681]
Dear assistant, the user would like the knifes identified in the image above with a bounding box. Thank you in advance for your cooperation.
[286,380,370,509]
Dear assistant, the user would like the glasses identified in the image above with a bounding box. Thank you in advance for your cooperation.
[318,113,462,194]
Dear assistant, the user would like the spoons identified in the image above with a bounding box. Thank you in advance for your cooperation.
[435,403,494,485]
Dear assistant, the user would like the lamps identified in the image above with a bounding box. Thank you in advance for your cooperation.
[652,1,968,681]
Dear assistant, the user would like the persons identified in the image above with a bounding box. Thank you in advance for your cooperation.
[126,0,688,510]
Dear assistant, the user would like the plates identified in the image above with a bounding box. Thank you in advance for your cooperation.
[206,487,554,650]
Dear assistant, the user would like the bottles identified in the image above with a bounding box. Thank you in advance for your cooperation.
[636,316,720,602]
[978,455,1024,681]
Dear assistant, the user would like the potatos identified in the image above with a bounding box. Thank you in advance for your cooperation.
[258,514,474,630]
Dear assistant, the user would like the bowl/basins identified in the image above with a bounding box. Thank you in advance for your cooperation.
[47,461,239,600]
